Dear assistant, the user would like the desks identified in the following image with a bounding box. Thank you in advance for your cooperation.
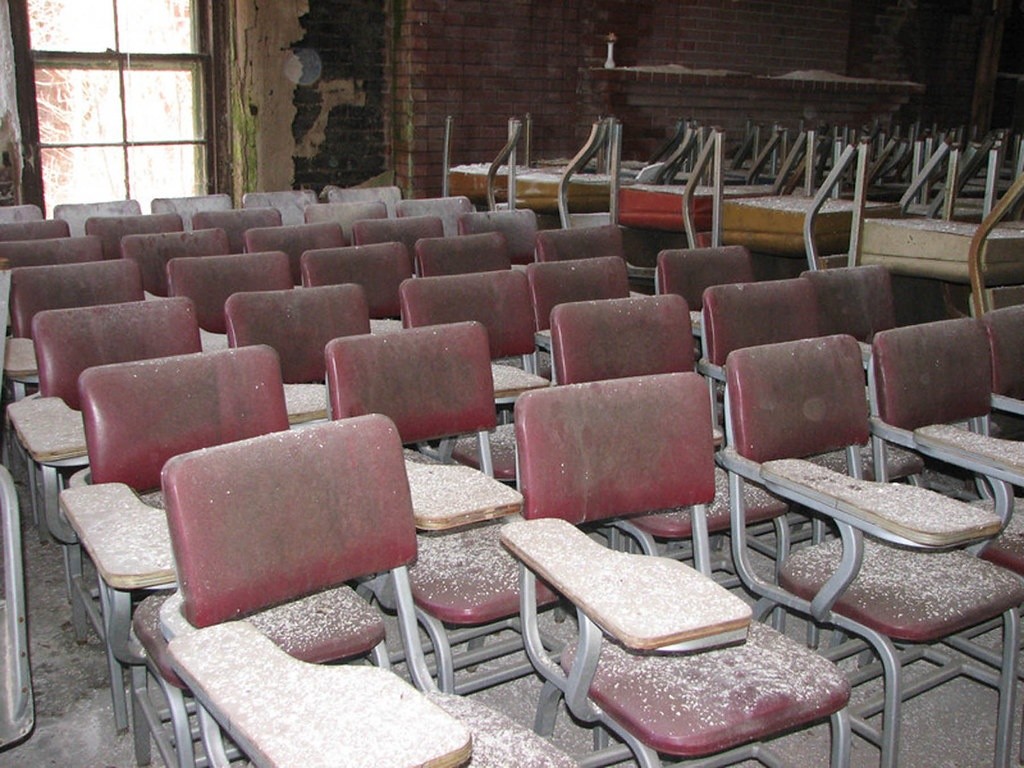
[167,620,474,768]
[284,383,330,429]
[59,479,178,589]
[492,363,549,404]
[761,458,1003,549]
[405,459,524,532]
[200,327,229,352]
[371,318,407,335]
[3,336,38,379]
[7,396,90,467]
[913,424,1024,487]
[500,517,753,652]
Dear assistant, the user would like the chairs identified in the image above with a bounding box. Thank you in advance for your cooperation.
[0,185,1024,768]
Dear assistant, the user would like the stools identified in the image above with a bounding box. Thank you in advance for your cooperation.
[442,114,1024,319]
